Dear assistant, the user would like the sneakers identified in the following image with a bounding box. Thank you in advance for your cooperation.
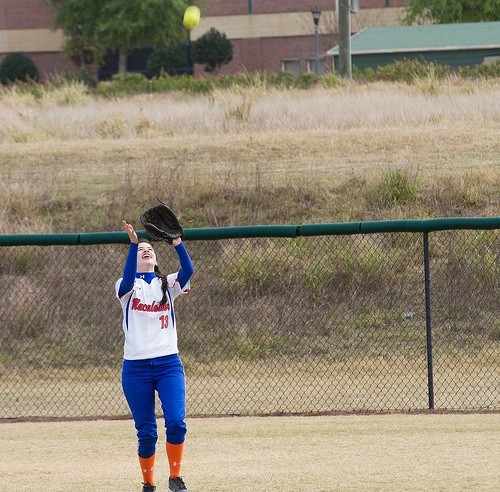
[142,482,156,492]
[169,476,188,492]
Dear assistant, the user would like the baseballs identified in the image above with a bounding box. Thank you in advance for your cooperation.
[183,5,201,28]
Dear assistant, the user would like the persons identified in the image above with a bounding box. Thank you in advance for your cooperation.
[115,219,195,492]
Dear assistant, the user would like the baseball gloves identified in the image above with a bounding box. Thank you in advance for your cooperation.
[140,203,184,246]
